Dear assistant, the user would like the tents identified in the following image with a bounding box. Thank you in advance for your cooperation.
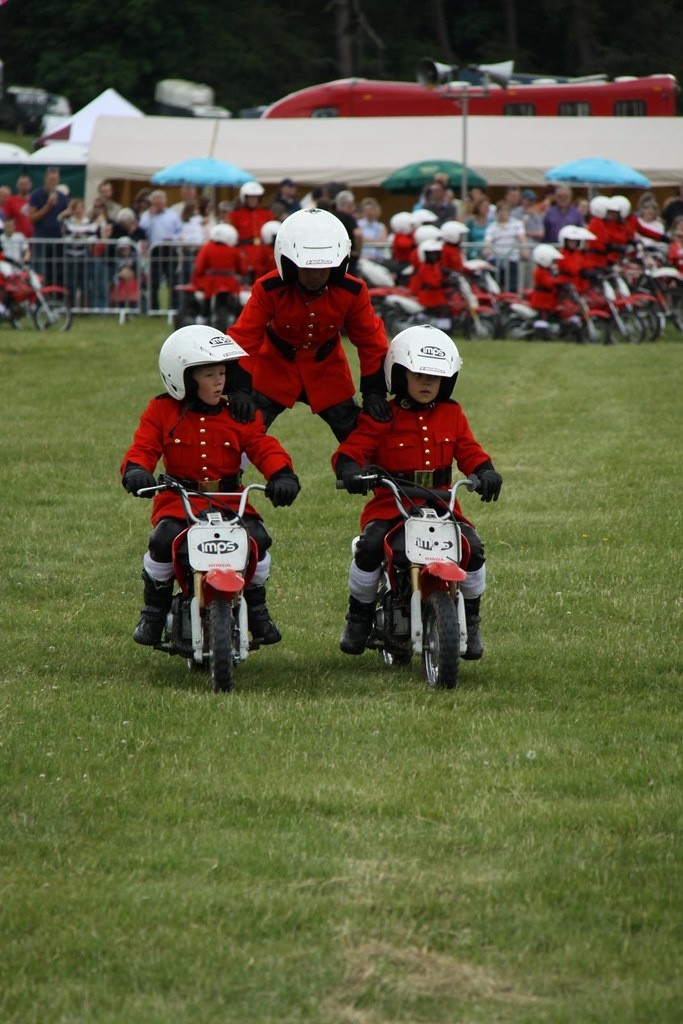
[85,115,683,210]
[33,88,145,150]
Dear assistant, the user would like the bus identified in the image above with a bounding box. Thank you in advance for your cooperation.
[260,72,683,118]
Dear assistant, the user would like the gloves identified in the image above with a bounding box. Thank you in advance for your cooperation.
[337,461,369,496]
[361,392,393,422]
[264,462,301,508]
[122,460,159,499]
[229,385,258,425]
[474,463,503,503]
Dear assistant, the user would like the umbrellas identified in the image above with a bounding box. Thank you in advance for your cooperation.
[544,157,651,197]
[151,159,254,187]
[381,160,485,190]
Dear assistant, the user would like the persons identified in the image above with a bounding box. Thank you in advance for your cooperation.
[331,325,503,661]
[0,167,683,336]
[120,325,301,646]
[226,208,392,487]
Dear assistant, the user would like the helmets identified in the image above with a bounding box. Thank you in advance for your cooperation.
[274,208,351,284]
[384,325,463,402]
[559,225,597,252]
[238,181,265,206]
[208,223,238,247]
[390,208,471,263]
[589,195,632,219]
[259,221,282,245]
[531,243,564,269]
[158,325,250,401]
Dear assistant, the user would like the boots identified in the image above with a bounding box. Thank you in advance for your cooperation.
[243,585,282,645]
[132,571,174,646]
[460,595,484,660]
[339,595,377,655]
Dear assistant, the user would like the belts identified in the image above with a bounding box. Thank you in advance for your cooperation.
[162,470,246,493]
[265,324,339,363]
[379,465,453,489]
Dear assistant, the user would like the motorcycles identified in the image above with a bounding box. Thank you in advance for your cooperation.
[172,238,683,345]
[335,465,482,690]
[137,473,268,692]
[0,255,72,332]
